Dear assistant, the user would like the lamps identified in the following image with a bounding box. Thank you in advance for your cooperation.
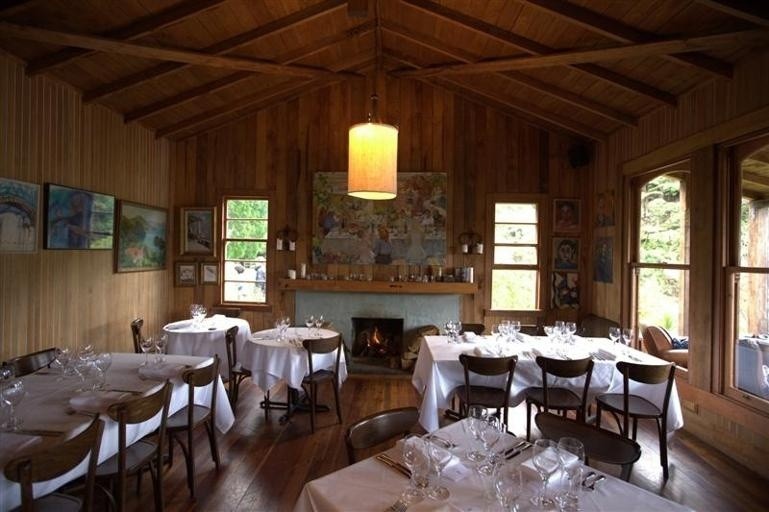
[347,0,399,201]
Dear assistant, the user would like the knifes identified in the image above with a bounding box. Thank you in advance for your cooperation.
[500,441,531,460]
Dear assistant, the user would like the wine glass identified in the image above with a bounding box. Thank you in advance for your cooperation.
[622,328,634,345]
[52,343,112,393]
[402,406,587,512]
[275,314,326,342]
[442,321,462,346]
[139,334,168,368]
[544,320,577,352]
[189,303,207,332]
[609,327,621,345]
[491,319,522,350]
[0,364,25,433]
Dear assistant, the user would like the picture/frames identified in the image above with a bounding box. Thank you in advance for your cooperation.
[551,237,581,272]
[113,199,169,273]
[0,176,41,255]
[552,198,582,233]
[43,182,116,250]
[174,261,198,288]
[200,262,221,285]
[179,206,218,260]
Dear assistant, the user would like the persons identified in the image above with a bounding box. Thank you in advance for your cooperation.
[554,239,575,268]
[358,201,445,265]
[558,202,577,226]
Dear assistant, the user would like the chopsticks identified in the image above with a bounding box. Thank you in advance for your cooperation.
[589,347,616,361]
[11,430,64,436]
[376,452,427,487]
[104,390,143,394]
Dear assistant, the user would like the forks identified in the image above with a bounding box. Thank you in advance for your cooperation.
[579,470,605,491]
